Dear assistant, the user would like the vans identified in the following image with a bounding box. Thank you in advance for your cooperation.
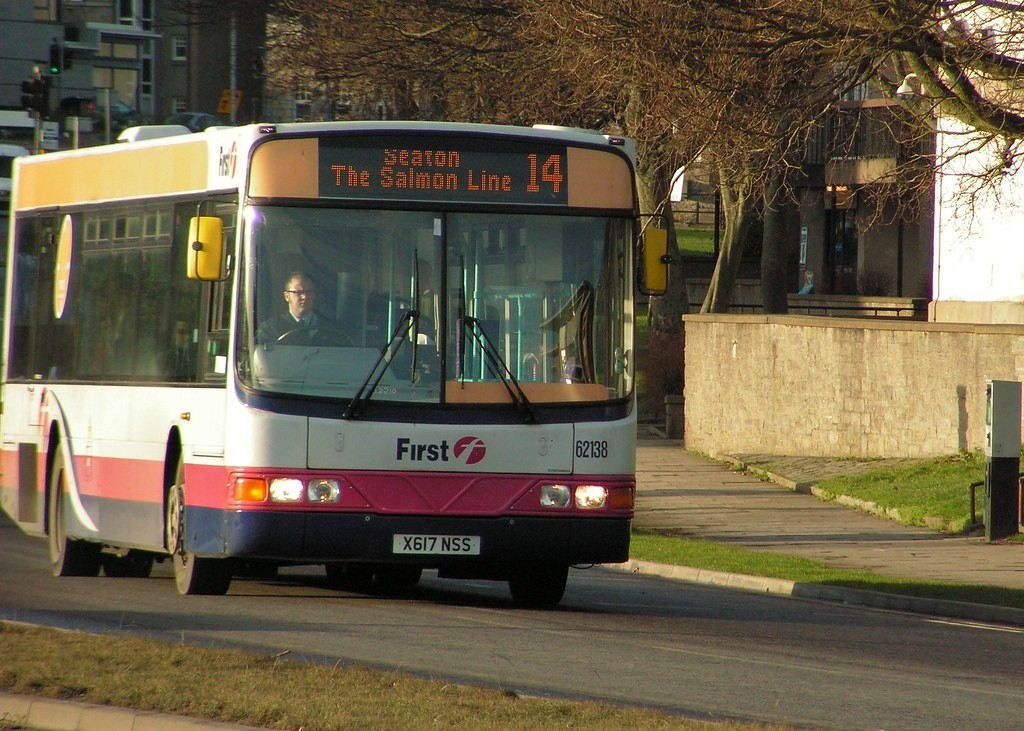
[0,145,31,215]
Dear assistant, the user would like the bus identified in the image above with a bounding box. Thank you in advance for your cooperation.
[0,109,36,152]
[0,115,674,602]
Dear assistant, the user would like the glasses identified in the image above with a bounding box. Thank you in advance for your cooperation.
[286,290,313,296]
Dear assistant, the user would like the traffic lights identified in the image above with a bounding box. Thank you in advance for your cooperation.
[49,42,63,76]
[61,96,96,115]
[20,79,44,117]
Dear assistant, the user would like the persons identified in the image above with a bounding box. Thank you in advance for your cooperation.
[259,272,341,345]
